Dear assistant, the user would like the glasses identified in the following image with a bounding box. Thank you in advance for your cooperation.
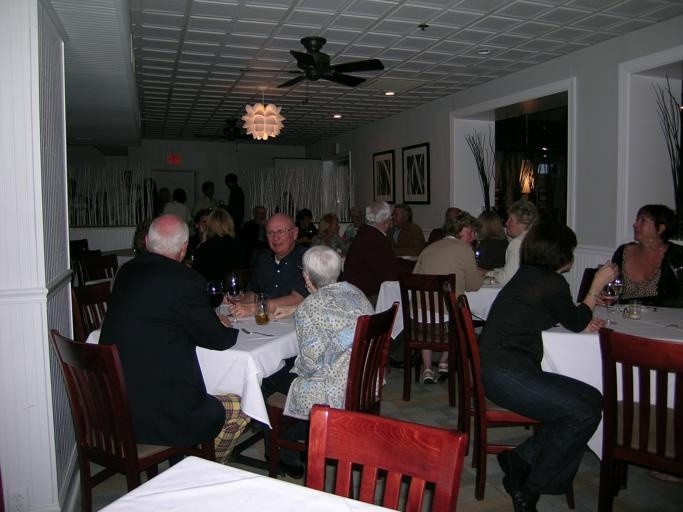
[266,226,294,238]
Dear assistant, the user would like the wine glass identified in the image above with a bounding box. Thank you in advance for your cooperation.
[225,272,245,325]
[205,281,223,312]
[598,283,619,328]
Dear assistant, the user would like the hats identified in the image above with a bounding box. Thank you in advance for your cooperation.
[352,207,363,217]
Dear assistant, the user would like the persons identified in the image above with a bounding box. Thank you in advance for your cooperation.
[132,191,542,300]
[612,204,682,309]
[261,244,386,479]
[479,222,619,511]
[95,213,251,466]
[70,170,245,227]
[223,212,310,316]
[408,210,484,384]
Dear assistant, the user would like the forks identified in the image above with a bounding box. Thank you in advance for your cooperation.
[241,328,274,337]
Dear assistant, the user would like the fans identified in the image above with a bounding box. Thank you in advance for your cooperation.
[275,34,387,106]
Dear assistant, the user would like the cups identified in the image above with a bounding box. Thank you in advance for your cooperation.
[253,293,270,326]
[628,300,640,320]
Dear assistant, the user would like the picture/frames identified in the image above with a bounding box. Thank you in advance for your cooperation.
[399,142,431,205]
[370,148,397,206]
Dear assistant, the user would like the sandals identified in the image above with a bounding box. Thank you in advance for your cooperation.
[423,368,438,384]
[439,363,449,374]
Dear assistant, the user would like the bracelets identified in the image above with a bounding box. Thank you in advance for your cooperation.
[588,292,598,299]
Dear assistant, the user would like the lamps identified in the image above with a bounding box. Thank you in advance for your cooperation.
[521,114,531,194]
[239,84,286,143]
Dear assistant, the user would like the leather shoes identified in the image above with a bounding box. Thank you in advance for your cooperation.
[497,450,529,479]
[279,459,304,479]
[503,473,539,512]
